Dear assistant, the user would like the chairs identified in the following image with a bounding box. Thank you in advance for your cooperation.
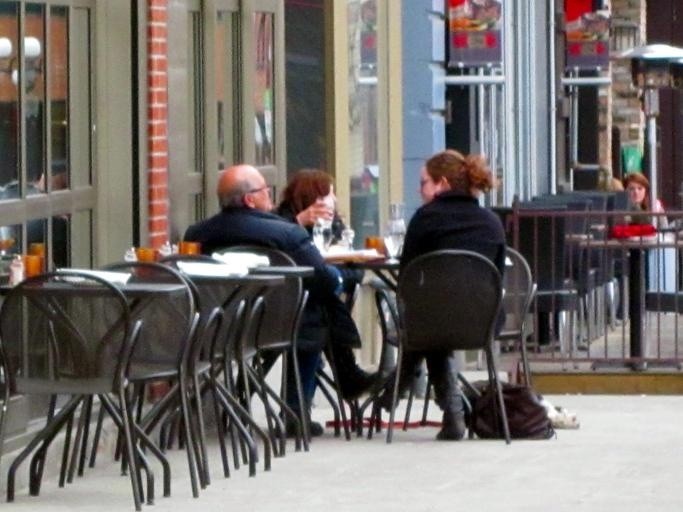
[367,250,511,443]
[104,245,316,510]
[501,247,536,390]
[1,270,145,512]
[512,192,632,351]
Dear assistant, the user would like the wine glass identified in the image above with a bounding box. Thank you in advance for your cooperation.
[313,223,330,255]
[385,221,404,263]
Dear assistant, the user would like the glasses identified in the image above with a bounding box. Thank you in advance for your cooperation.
[246,185,272,194]
[418,178,434,188]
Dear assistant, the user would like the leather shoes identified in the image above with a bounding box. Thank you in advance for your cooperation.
[275,407,326,436]
[334,365,390,400]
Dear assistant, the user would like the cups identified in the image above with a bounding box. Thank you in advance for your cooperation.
[23,256,43,277]
[178,242,200,257]
[365,237,384,260]
[317,198,334,227]
[134,249,157,277]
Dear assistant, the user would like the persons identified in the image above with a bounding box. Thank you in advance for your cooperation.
[183,165,389,437]
[378,150,507,440]
[625,174,668,231]
[597,178,650,225]
[270,170,348,246]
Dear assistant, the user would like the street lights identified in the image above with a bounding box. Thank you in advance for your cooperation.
[611,43,682,230]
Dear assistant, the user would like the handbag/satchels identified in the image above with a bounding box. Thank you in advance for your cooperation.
[466,383,554,440]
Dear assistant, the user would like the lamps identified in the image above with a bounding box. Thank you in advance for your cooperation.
[0,36,43,91]
[616,44,682,118]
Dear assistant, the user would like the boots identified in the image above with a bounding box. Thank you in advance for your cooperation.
[434,374,467,441]
[380,361,419,412]
